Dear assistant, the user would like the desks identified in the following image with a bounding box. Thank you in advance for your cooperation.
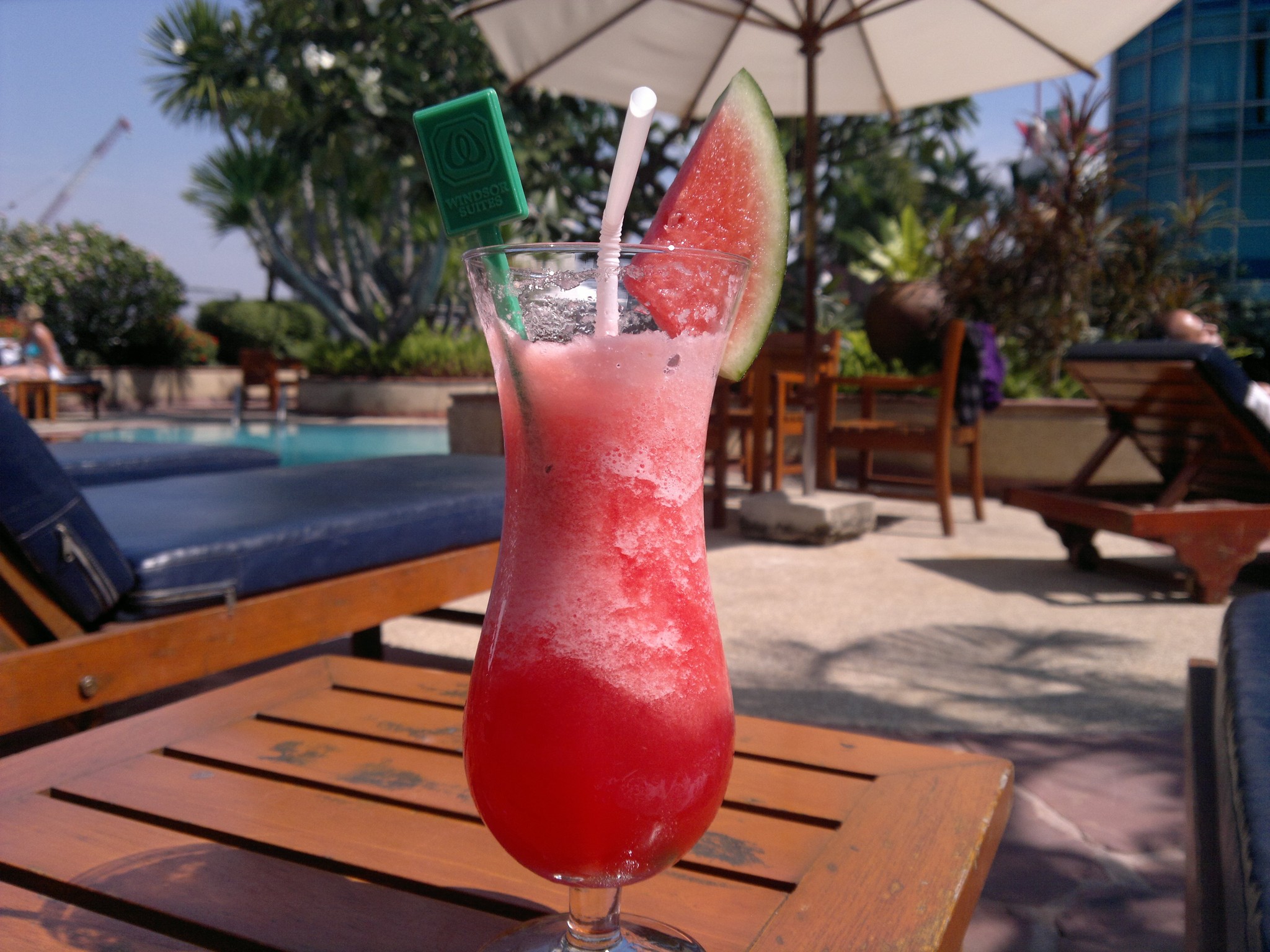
[0,652,1017,952]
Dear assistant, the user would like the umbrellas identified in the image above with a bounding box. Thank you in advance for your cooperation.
[446,0,1174,493]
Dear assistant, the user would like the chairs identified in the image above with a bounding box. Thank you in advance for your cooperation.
[701,318,842,533]
[999,341,1270,606]
[1,318,505,739]
[814,319,984,539]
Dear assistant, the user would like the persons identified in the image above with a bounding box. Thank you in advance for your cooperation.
[0,302,71,382]
[1136,308,1270,428]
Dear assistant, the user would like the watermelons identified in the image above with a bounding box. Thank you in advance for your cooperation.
[625,69,792,384]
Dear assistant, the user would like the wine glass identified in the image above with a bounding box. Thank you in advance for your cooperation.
[462,241,735,952]
